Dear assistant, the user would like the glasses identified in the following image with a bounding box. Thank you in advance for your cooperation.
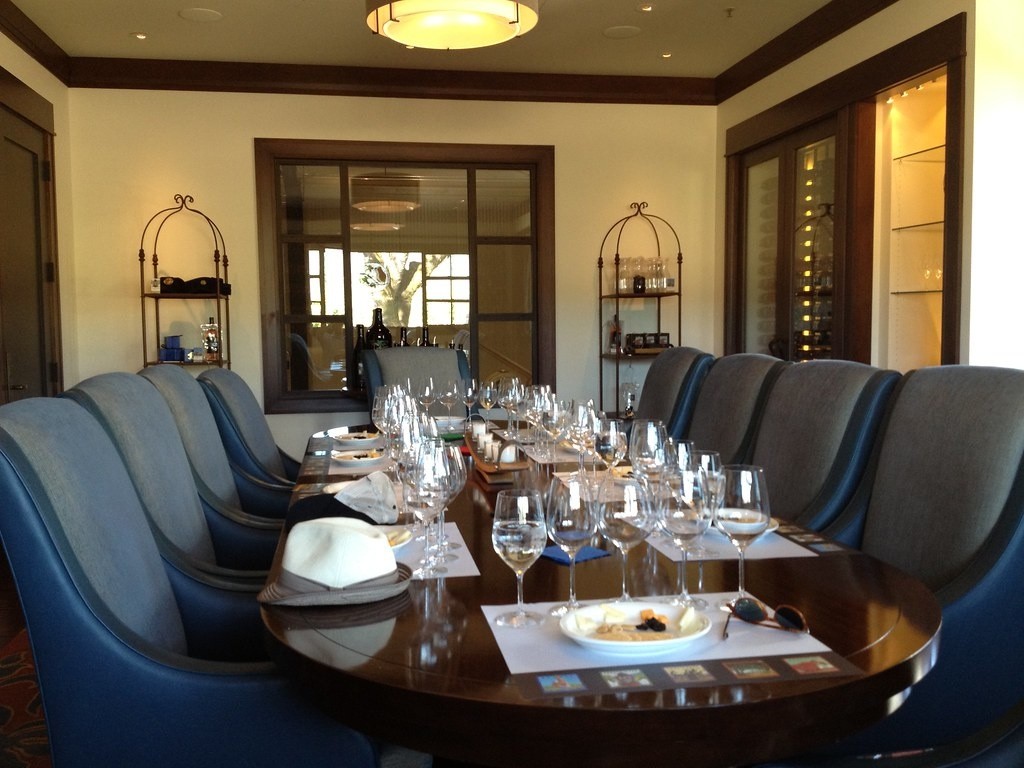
[722,597,810,641]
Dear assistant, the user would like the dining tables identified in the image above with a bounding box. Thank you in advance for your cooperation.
[258,417,942,712]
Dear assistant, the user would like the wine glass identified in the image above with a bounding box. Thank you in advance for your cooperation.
[374,376,769,627]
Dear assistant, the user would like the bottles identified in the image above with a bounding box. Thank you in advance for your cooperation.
[616,256,675,293]
[205,316,218,361]
[352,308,463,396]
[608,315,621,349]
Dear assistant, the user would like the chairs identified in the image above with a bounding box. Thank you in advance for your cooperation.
[0,346,1024,767]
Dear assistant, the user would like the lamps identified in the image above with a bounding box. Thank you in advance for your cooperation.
[365,0,540,51]
[350,208,407,232]
[350,168,421,214]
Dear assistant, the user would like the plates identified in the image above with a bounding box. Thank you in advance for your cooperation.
[322,481,358,494]
[608,465,658,485]
[559,602,711,653]
[433,414,465,426]
[331,450,385,466]
[707,506,779,537]
[333,432,380,445]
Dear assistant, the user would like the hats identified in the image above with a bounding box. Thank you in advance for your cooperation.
[260,592,410,672]
[256,518,412,607]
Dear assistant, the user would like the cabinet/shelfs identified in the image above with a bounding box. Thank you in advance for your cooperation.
[137,191,232,377]
[597,199,683,424]
[738,66,948,375]
